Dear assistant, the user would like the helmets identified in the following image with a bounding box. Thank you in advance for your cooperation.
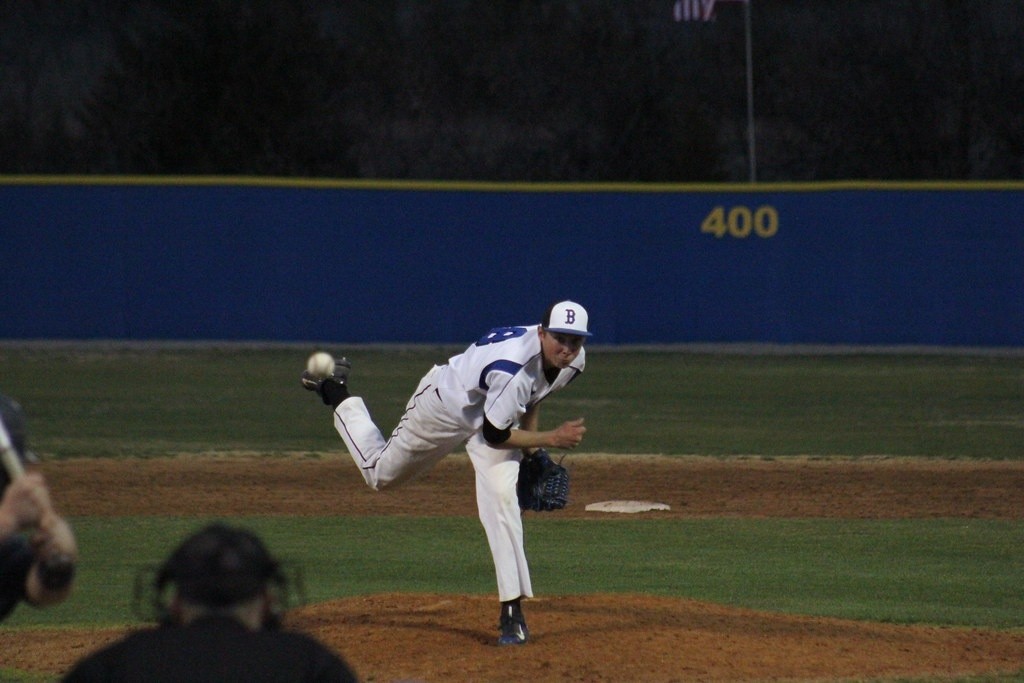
[166,523,273,619]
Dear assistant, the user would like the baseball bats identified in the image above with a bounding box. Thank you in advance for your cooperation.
[0,417,77,554]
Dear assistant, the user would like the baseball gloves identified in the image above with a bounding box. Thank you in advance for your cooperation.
[516,448,571,512]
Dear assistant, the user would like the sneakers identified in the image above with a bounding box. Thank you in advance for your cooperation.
[497,613,529,644]
[301,360,352,395]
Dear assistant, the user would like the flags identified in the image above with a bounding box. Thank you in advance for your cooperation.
[674,0,716,22]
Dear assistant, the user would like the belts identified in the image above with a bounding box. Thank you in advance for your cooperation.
[436,387,443,401]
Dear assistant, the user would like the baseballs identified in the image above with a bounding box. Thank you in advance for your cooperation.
[307,351,337,379]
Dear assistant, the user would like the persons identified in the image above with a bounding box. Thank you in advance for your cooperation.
[302,301,593,646]
[0,394,76,623]
[60,525,356,683]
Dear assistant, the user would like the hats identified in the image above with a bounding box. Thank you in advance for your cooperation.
[542,298,592,336]
